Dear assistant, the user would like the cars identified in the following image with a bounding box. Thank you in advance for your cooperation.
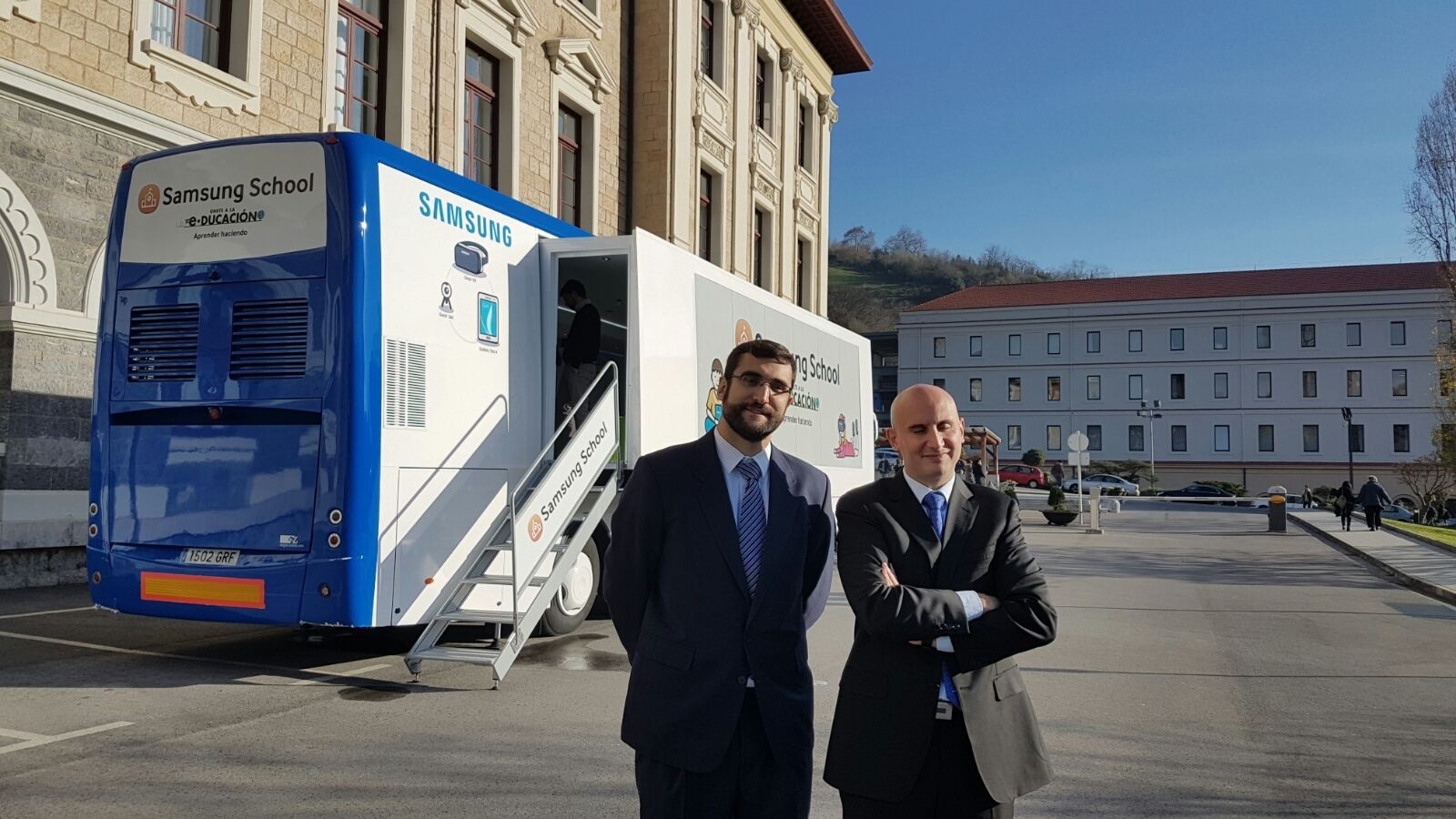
[988,465,1044,488]
[1250,491,1317,511]
[1065,475,1140,496]
[1366,504,1414,523]
[875,446,905,470]
[1157,484,1235,507]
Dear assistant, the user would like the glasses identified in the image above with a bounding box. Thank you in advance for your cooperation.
[728,373,794,393]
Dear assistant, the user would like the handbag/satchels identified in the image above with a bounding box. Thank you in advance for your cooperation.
[1337,495,1346,508]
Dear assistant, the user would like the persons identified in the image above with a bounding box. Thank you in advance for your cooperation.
[957,457,987,484]
[893,458,902,475]
[602,339,836,819]
[559,279,601,431]
[823,383,1058,819]
[1302,484,1311,496]
[1334,480,1356,531]
[1356,475,1392,531]
[1302,488,1313,509]
[877,454,891,479]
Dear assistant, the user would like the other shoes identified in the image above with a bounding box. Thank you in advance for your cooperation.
[1347,528,1349,531]
[1376,527,1378,530]
[1370,528,1374,531]
[1342,527,1345,530]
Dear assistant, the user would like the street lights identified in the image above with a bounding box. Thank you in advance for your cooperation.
[1343,406,1353,490]
[1140,399,1162,490]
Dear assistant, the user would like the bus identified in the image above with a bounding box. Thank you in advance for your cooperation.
[83,135,867,687]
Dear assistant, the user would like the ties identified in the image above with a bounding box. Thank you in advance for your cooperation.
[922,490,961,708]
[736,459,767,601]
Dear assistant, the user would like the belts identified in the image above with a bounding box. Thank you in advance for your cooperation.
[936,700,964,721]
